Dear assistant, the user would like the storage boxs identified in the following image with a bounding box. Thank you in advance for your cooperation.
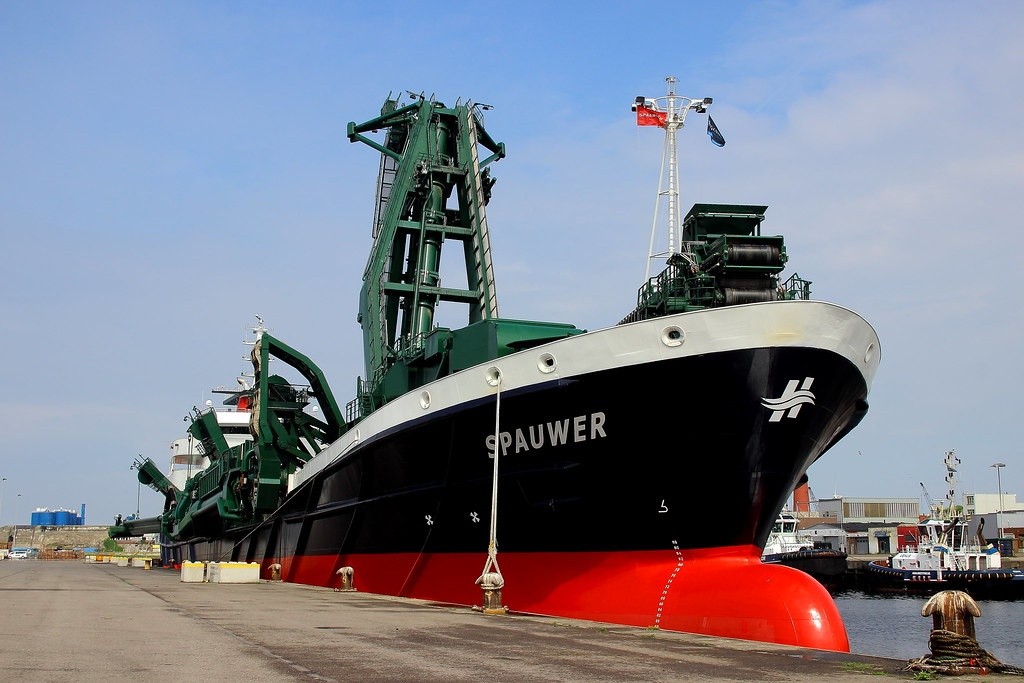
[42,550,84,559]
[207,561,260,584]
[181,560,204,582]
[0,542,11,561]
[86,555,152,568]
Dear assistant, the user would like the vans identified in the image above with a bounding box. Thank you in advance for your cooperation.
[8,551,27,559]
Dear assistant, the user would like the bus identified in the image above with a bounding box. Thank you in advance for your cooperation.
[73,548,99,553]
[14,548,39,556]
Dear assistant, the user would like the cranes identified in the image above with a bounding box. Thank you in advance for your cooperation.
[920,482,937,511]
[808,487,819,512]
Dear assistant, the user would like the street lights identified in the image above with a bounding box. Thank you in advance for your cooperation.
[13,493,22,546]
[990,462,1006,538]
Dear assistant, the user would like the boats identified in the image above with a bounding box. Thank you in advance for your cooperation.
[867,448,1024,599]
[109,77,881,647]
[761,505,849,592]
